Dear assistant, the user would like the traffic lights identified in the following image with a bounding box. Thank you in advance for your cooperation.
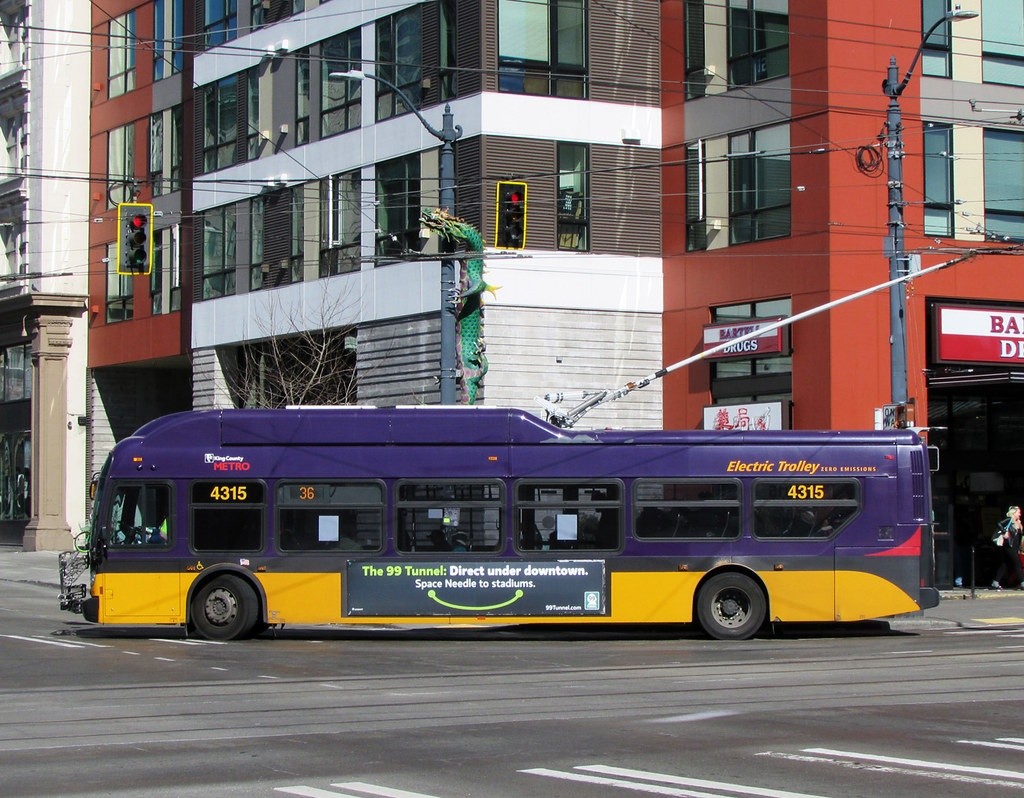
[495,182,525,249]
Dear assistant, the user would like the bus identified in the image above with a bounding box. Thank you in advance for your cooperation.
[58,250,980,645]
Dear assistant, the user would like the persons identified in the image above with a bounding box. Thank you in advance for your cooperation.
[591,491,618,549]
[143,491,168,545]
[953,494,977,588]
[989,505,1024,591]
[691,491,738,537]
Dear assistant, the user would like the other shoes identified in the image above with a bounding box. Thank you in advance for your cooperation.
[989,584,1003,592]
[1017,584,1024,591]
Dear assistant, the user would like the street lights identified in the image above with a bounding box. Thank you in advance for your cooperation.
[327,68,461,406]
[886,11,977,422]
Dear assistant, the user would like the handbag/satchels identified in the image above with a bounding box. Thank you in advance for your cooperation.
[994,517,1012,547]
